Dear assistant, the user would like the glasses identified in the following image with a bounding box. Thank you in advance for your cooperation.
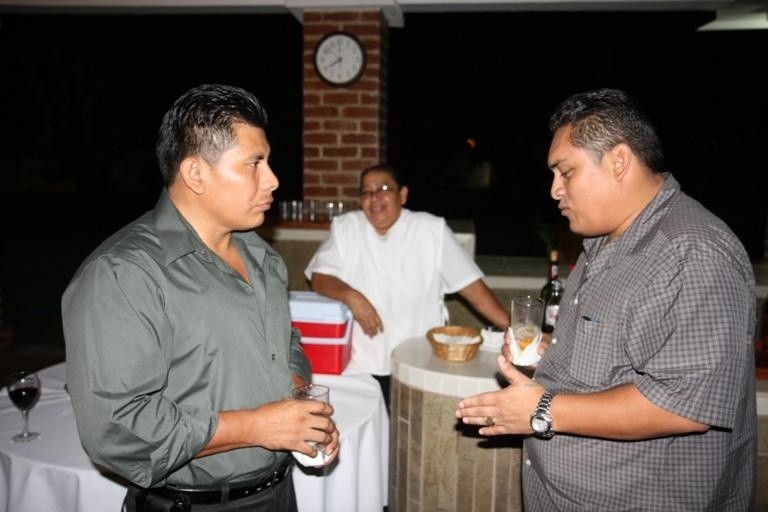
[361,184,398,196]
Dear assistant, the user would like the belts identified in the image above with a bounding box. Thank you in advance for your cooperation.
[149,454,295,505]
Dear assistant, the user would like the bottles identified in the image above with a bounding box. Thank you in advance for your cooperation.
[539,249,564,336]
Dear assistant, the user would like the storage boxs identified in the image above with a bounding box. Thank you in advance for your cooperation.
[288,290,352,375]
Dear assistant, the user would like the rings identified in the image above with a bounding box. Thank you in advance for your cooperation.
[486,416,495,425]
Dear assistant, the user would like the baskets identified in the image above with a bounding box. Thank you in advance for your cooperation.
[428,326,483,363]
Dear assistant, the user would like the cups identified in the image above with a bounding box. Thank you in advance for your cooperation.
[290,384,330,451]
[508,295,545,354]
[277,199,347,224]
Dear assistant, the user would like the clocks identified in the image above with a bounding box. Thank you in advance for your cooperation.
[314,33,365,88]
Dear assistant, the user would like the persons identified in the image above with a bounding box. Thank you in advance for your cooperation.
[454,86,760,512]
[59,78,342,511]
[304,163,511,414]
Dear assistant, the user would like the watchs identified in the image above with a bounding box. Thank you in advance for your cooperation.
[529,392,557,440]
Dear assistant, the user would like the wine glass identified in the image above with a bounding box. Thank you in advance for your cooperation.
[6,369,42,444]
